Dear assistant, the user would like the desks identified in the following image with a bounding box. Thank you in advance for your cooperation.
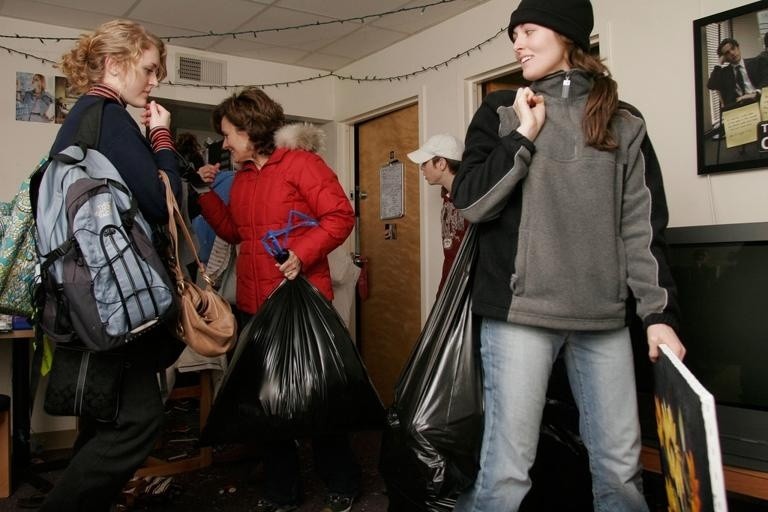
[0,328,214,498]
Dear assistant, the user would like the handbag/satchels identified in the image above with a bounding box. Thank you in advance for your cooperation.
[44,347,121,422]
[179,275,239,356]
[0,158,43,317]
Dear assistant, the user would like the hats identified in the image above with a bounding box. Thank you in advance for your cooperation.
[508,0,593,52]
[407,135,465,165]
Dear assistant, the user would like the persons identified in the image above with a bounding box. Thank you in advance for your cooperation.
[450,0,685,511]
[706,32,768,105]
[408,132,470,298]
[192,86,356,512]
[16,73,53,122]
[41,19,183,512]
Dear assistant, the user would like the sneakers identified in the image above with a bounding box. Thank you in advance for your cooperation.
[325,493,355,512]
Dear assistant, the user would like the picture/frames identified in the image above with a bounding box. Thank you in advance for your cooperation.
[692,0,767,175]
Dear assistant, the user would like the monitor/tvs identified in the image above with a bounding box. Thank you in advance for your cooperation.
[628,222,768,473]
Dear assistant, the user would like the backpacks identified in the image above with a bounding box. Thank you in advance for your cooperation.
[29,99,178,353]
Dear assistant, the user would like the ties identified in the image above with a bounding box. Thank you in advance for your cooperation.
[735,66,745,95]
